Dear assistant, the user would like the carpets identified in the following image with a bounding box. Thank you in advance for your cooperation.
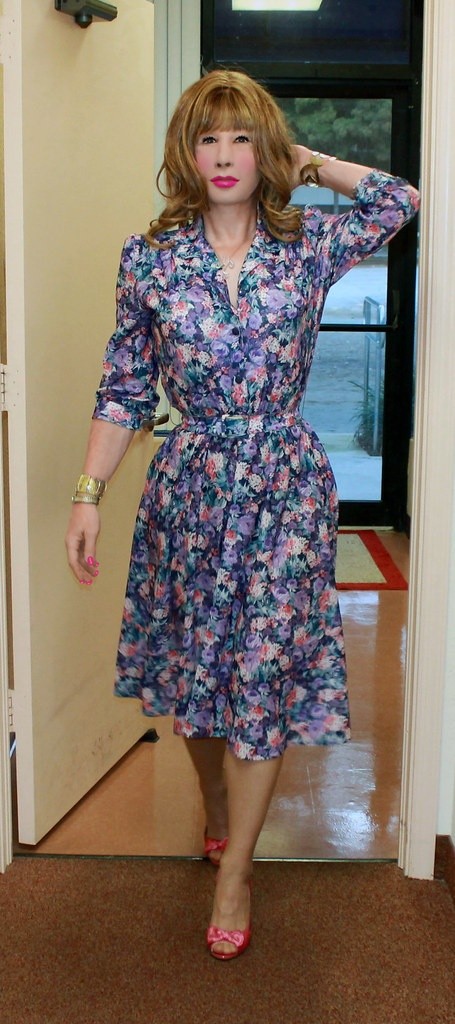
[335,530,409,590]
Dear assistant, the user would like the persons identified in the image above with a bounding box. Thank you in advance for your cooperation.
[68,69,419,961]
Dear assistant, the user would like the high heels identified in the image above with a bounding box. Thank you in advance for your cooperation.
[206,870,258,960]
[204,825,230,865]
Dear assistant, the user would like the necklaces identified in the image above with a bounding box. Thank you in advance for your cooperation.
[205,224,256,275]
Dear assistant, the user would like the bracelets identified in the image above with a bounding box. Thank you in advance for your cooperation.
[69,495,99,505]
[75,474,107,497]
[301,149,336,188]
